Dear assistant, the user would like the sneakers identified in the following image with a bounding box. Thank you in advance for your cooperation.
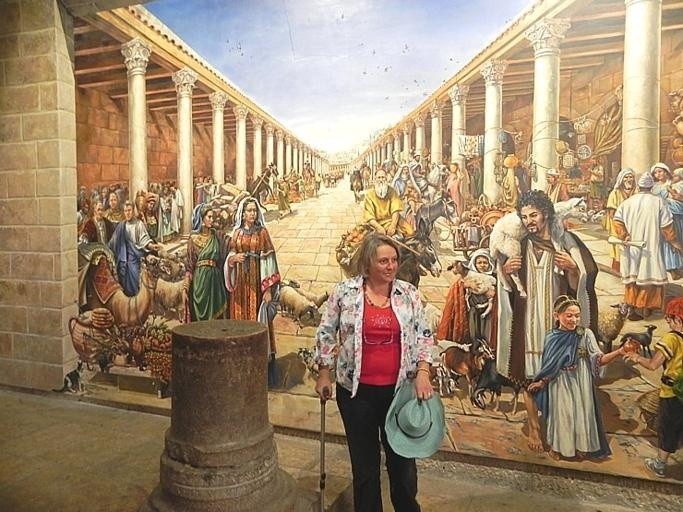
[642,455,669,479]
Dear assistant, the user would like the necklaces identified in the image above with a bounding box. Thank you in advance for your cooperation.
[362,281,395,309]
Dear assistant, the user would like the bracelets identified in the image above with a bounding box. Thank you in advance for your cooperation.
[416,366,431,376]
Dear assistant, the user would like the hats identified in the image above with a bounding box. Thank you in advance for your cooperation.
[385,380,446,459]
[665,295,683,321]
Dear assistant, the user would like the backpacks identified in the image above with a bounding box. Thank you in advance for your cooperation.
[669,330,683,404]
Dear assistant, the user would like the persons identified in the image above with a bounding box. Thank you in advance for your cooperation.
[347,149,438,276]
[314,234,438,512]
